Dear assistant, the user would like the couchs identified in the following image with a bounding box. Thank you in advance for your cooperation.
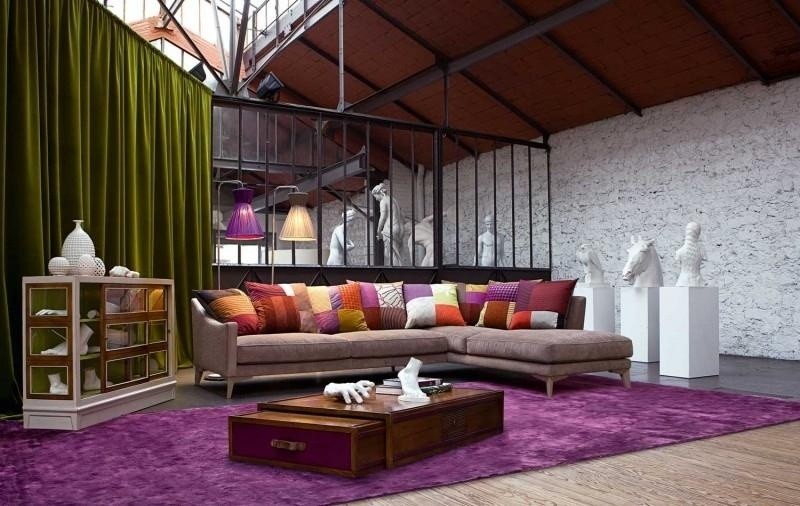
[191,297,633,398]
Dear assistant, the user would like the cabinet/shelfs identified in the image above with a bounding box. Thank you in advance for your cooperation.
[228,386,504,482]
[22,276,176,433]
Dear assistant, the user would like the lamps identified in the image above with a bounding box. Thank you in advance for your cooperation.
[190,60,207,82]
[256,71,285,100]
[205,180,267,380]
[272,186,317,286]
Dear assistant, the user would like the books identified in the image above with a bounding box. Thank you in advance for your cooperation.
[375,379,453,387]
[375,383,452,396]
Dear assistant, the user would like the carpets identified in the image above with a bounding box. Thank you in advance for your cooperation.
[1,373,800,506]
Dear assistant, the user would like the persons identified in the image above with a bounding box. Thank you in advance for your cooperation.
[82,368,115,393]
[35,308,69,317]
[322,379,375,403]
[398,357,429,400]
[39,323,95,358]
[405,212,447,266]
[44,371,69,396]
[474,215,508,267]
[326,208,356,265]
[371,183,405,267]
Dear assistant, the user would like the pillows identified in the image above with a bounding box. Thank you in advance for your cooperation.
[242,280,307,332]
[307,282,369,333]
[474,279,544,329]
[441,279,488,325]
[346,280,406,329]
[403,283,466,328]
[507,277,580,329]
[192,287,259,336]
